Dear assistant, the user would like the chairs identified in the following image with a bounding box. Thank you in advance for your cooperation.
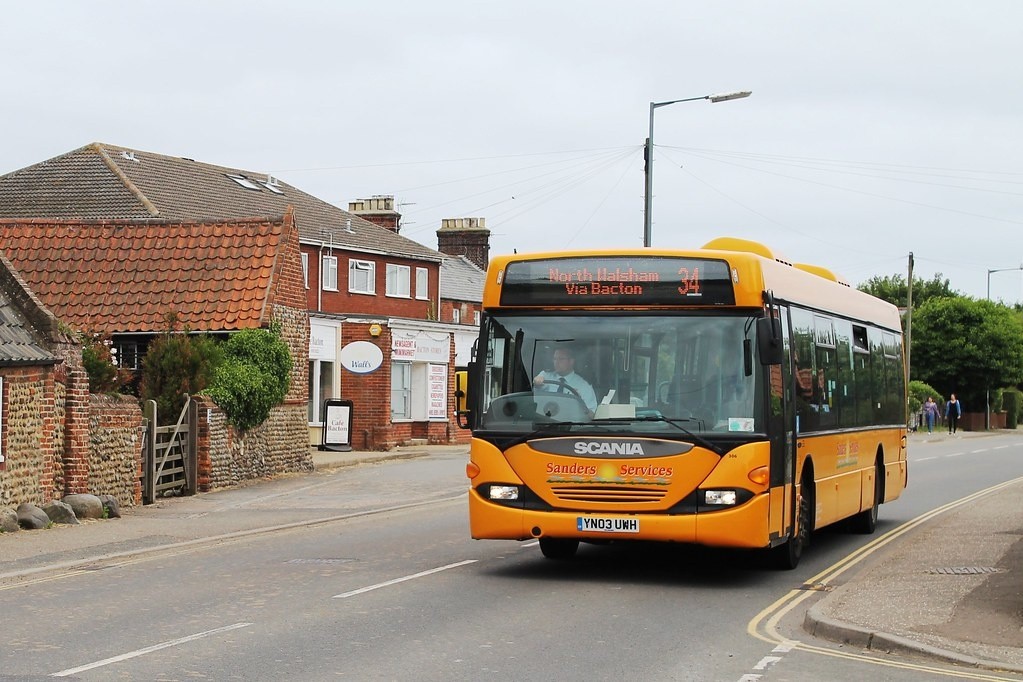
[575,369,600,406]
[705,373,746,406]
[666,374,701,409]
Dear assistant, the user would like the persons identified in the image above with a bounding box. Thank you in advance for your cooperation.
[533,346,597,420]
[945,394,961,435]
[923,396,940,434]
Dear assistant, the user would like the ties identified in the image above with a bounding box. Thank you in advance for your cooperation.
[557,378,565,394]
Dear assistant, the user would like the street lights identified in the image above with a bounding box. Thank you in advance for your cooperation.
[643,90,752,247]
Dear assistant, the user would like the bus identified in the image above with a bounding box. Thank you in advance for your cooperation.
[455,236,908,569]
